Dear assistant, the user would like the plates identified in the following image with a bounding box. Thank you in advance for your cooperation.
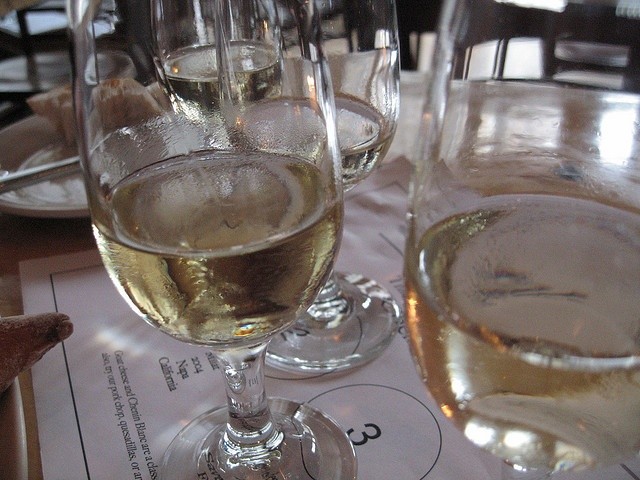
[0,112,114,223]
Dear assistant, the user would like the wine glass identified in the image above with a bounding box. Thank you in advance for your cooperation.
[56,0,359,479]
[399,1,640,480]
[143,0,286,135]
[211,0,400,382]
